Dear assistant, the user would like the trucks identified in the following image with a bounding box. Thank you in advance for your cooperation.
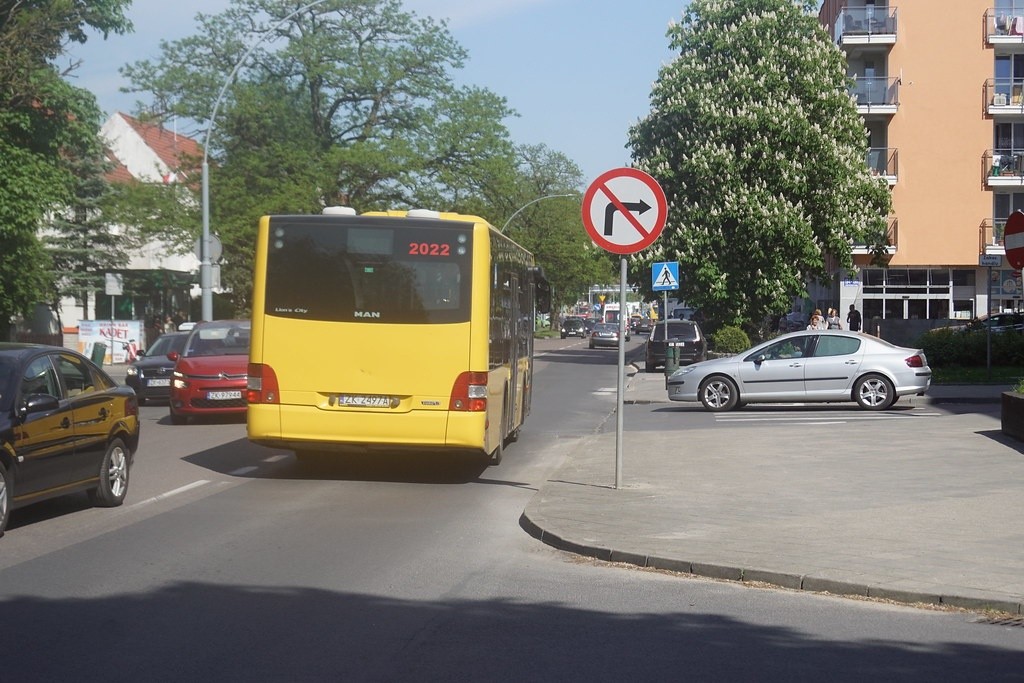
[602,301,641,342]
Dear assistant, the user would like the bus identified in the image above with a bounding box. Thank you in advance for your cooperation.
[670,308,699,320]
[650,305,658,320]
[245,207,552,466]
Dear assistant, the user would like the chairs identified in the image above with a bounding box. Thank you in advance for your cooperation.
[21,366,41,394]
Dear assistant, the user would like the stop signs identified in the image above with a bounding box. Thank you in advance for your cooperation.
[1004,210,1024,269]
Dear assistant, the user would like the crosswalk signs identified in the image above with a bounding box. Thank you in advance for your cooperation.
[652,262,680,291]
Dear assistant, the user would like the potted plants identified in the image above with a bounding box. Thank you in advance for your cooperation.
[998,224,1005,245]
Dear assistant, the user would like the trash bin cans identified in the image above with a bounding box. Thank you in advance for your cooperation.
[664,338,683,378]
[90,342,107,368]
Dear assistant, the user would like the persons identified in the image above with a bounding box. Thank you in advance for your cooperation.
[826,308,842,329]
[806,316,823,331]
[679,314,687,321]
[810,309,825,327]
[847,304,861,332]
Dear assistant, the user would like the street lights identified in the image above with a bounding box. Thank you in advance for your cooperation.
[500,194,578,234]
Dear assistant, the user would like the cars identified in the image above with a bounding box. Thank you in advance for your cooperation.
[167,319,251,425]
[929,312,1024,353]
[589,323,620,349]
[646,320,706,373]
[667,329,932,412]
[631,314,653,334]
[0,342,141,536]
[560,317,602,339]
[125,322,198,407]
